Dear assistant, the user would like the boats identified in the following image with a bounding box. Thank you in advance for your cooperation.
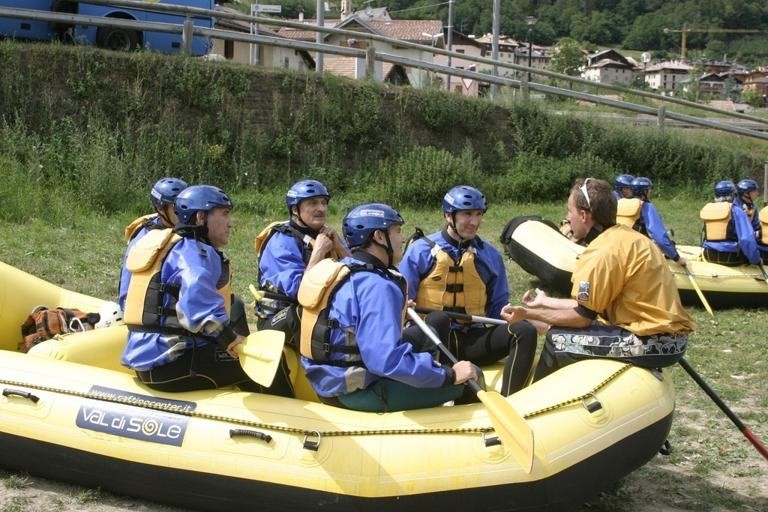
[1,248,682,510]
[498,209,768,312]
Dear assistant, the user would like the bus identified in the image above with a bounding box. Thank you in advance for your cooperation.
[1,0,220,59]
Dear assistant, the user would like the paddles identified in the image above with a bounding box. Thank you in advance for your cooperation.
[232,330,287,388]
[681,265,715,317]
[404,305,534,477]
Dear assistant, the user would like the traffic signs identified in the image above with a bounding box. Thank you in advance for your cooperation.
[250,3,284,14]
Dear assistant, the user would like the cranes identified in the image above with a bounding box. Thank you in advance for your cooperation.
[661,21,761,62]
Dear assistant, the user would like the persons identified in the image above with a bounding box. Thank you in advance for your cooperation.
[119,177,250,337]
[396,184,548,397]
[121,184,295,398]
[613,174,634,200]
[297,203,487,413]
[736,179,768,264]
[615,177,687,266]
[500,177,696,389]
[700,180,764,267]
[252,179,352,348]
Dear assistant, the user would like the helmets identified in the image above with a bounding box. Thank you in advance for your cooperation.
[150,176,232,225]
[442,185,489,217]
[342,202,404,249]
[714,179,737,195]
[286,179,331,211]
[737,178,760,193]
[615,174,654,189]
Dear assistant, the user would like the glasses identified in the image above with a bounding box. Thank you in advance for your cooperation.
[580,177,596,210]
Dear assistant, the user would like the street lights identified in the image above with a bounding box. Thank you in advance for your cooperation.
[636,50,651,103]
[522,13,539,97]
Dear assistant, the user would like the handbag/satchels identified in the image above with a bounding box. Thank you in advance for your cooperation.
[20,306,100,352]
